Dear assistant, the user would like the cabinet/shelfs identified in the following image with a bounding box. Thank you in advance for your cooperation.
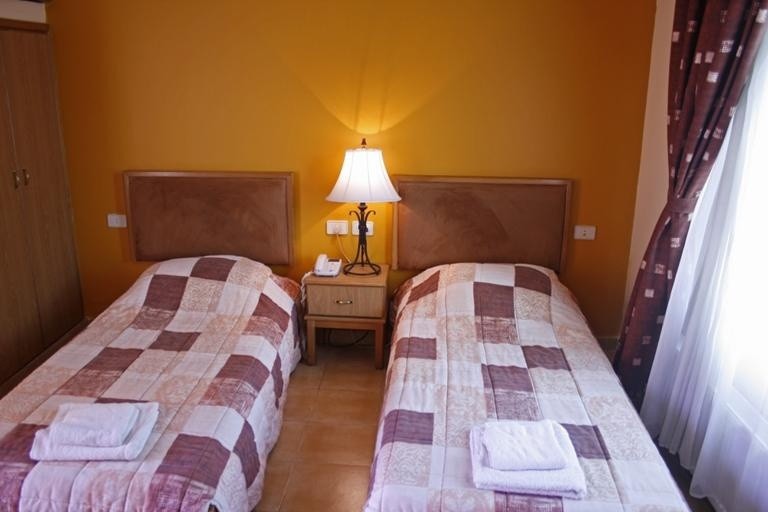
[0,27,83,384]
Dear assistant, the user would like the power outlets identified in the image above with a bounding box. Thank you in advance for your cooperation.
[574,225,596,240]
[326,220,348,234]
[107,213,127,228]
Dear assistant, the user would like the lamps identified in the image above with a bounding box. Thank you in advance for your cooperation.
[324,138,401,276]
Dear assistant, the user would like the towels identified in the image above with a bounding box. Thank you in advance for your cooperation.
[30,401,159,460]
[482,419,564,471]
[469,421,585,500]
[53,404,139,445]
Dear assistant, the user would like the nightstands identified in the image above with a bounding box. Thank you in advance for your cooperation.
[304,263,389,370]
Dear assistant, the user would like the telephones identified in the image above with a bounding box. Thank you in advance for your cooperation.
[314,254,342,277]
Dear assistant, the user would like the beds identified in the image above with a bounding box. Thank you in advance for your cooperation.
[0,169,302,512]
[362,175,692,512]
